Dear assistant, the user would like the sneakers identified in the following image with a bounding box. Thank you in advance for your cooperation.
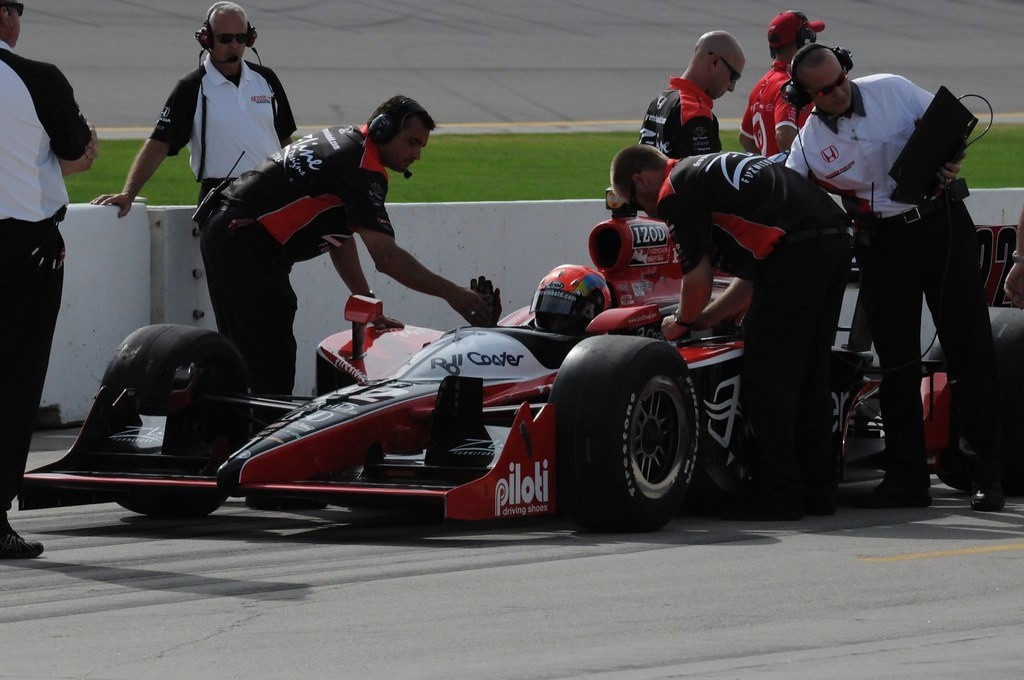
[0,534,44,558]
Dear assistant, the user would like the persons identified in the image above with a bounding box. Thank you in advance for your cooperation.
[785,44,1007,512]
[738,9,826,167]
[0,0,97,562]
[91,1,296,233]
[635,31,747,161]
[1004,206,1024,310]
[610,144,858,520]
[200,94,493,509]
[470,264,611,369]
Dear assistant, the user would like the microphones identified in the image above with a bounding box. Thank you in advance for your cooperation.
[206,49,238,64]
[403,169,413,179]
[803,108,830,118]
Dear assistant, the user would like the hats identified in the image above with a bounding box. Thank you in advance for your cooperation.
[769,12,825,48]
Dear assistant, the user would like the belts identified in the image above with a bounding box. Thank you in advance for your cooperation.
[782,224,849,245]
[865,194,945,231]
[216,204,253,216]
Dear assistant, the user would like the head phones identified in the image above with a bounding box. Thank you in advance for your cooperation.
[195,3,257,50]
[367,101,426,145]
[794,11,817,49]
[785,43,854,111]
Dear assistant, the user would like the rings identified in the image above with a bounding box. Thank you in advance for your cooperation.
[470,310,476,315]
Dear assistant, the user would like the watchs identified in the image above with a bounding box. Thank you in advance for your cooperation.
[674,308,691,328]
[1011,250,1024,262]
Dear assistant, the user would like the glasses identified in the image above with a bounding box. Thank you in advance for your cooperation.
[628,169,644,212]
[212,32,251,44]
[785,10,808,33]
[709,51,741,83]
[803,66,848,95]
[0,3,24,16]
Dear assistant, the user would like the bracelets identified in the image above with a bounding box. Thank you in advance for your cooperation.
[365,290,373,298]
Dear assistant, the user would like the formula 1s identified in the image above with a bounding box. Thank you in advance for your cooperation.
[17,187,1024,536]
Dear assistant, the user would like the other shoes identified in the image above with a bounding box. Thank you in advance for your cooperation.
[806,499,833,514]
[972,484,1005,510]
[721,498,804,520]
[856,484,931,506]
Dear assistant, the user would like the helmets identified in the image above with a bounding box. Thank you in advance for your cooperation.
[530,264,610,335]
